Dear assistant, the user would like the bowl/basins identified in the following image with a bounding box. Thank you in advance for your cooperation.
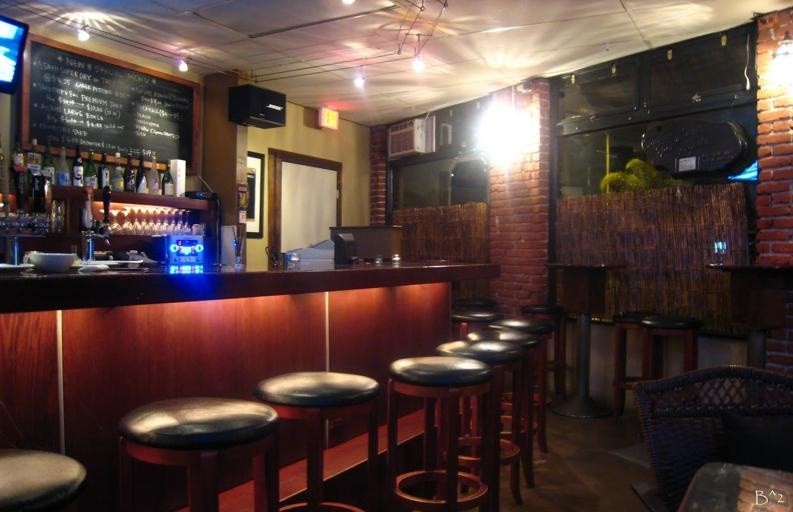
[29,253,77,273]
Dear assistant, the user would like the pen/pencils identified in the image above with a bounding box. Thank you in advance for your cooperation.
[232,226,245,257]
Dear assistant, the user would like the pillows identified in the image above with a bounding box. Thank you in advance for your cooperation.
[722,407,792,473]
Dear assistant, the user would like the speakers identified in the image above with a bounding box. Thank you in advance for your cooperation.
[228,84,288,129]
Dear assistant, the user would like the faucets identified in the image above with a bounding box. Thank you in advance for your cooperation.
[81,229,113,261]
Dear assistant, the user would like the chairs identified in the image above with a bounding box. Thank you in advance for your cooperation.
[635,365,792,512]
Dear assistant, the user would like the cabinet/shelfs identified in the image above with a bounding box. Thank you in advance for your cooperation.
[1,143,219,266]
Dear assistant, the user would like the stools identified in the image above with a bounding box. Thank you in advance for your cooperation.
[1,446,88,512]
[254,370,382,512]
[116,394,285,511]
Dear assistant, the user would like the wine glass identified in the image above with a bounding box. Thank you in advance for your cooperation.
[110,203,192,235]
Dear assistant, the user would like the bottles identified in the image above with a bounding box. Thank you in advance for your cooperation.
[98,151,110,189]
[11,143,26,181]
[200,223,208,235]
[161,159,175,196]
[148,152,160,195]
[136,152,147,193]
[124,153,136,192]
[9,235,20,265]
[84,149,98,190]
[26,136,41,177]
[72,144,83,187]
[85,228,96,260]
[56,146,71,186]
[111,152,124,191]
[42,136,56,186]
[192,224,200,234]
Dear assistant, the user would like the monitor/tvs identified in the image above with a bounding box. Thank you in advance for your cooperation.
[0,14,31,94]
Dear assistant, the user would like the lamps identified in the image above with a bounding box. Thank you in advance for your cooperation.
[176,52,190,73]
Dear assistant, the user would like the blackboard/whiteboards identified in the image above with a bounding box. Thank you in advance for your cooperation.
[20,31,202,175]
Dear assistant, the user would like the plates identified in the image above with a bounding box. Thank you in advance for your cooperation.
[0,263,35,270]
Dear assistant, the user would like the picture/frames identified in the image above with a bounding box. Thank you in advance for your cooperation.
[246,150,266,239]
